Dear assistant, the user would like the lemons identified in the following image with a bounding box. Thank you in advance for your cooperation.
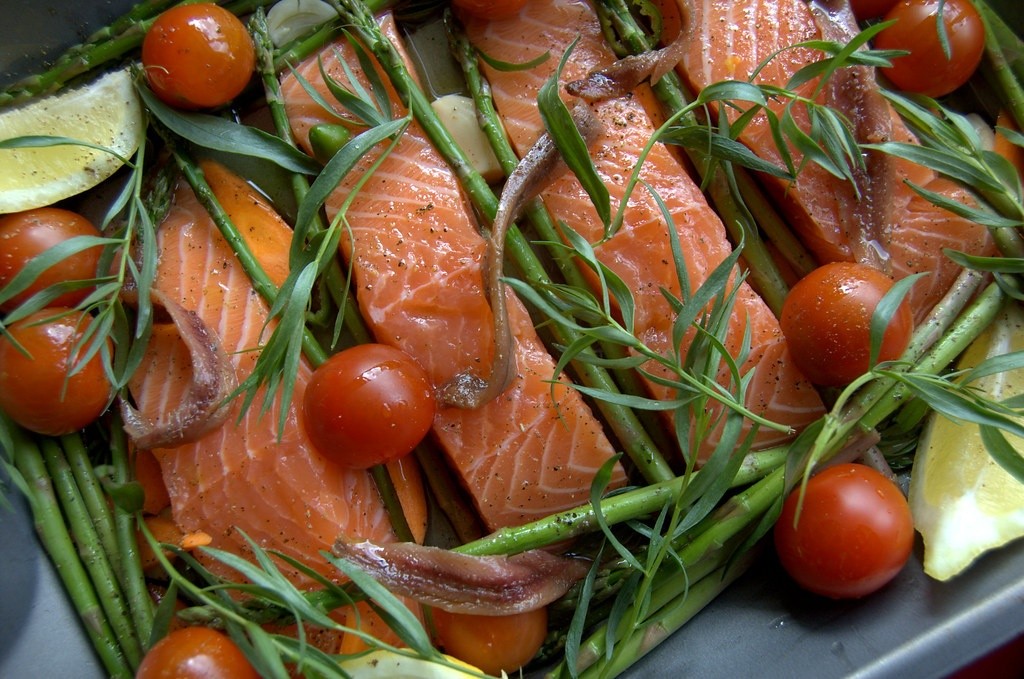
[0,62,152,215]
[909,292,1024,580]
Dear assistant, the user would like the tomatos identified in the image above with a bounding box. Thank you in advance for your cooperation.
[0,306,115,434]
[872,0,986,97]
[302,343,435,468]
[452,0,529,21]
[135,626,263,679]
[0,207,107,308]
[142,3,254,107]
[779,260,913,385]
[432,605,546,676]
[773,462,913,597]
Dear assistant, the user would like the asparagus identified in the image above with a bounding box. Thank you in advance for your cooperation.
[249,3,372,344]
[593,0,791,315]
[128,59,329,367]
[174,444,780,624]
[0,0,265,111]
[441,0,658,425]
[325,0,677,481]
[538,267,1019,607]
